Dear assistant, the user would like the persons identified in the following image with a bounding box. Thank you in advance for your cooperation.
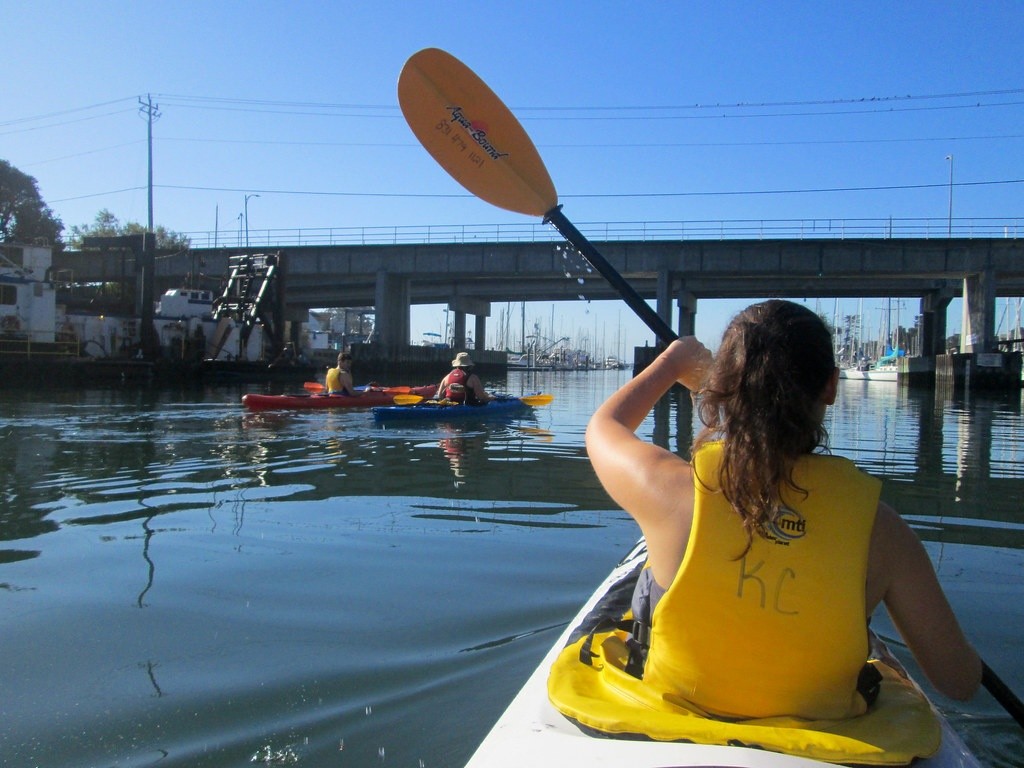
[326,353,369,397]
[583,297,984,722]
[438,352,489,405]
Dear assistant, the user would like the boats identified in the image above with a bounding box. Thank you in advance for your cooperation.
[242,383,438,412]
[369,392,542,422]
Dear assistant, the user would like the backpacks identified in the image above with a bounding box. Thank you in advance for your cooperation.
[445,369,468,401]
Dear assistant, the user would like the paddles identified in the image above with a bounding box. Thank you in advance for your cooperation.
[304,382,412,397]
[392,394,555,406]
[395,46,1024,729]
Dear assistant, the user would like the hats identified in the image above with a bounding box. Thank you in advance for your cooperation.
[452,353,475,367]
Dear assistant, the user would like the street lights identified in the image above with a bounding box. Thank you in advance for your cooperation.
[244,193,260,248]
